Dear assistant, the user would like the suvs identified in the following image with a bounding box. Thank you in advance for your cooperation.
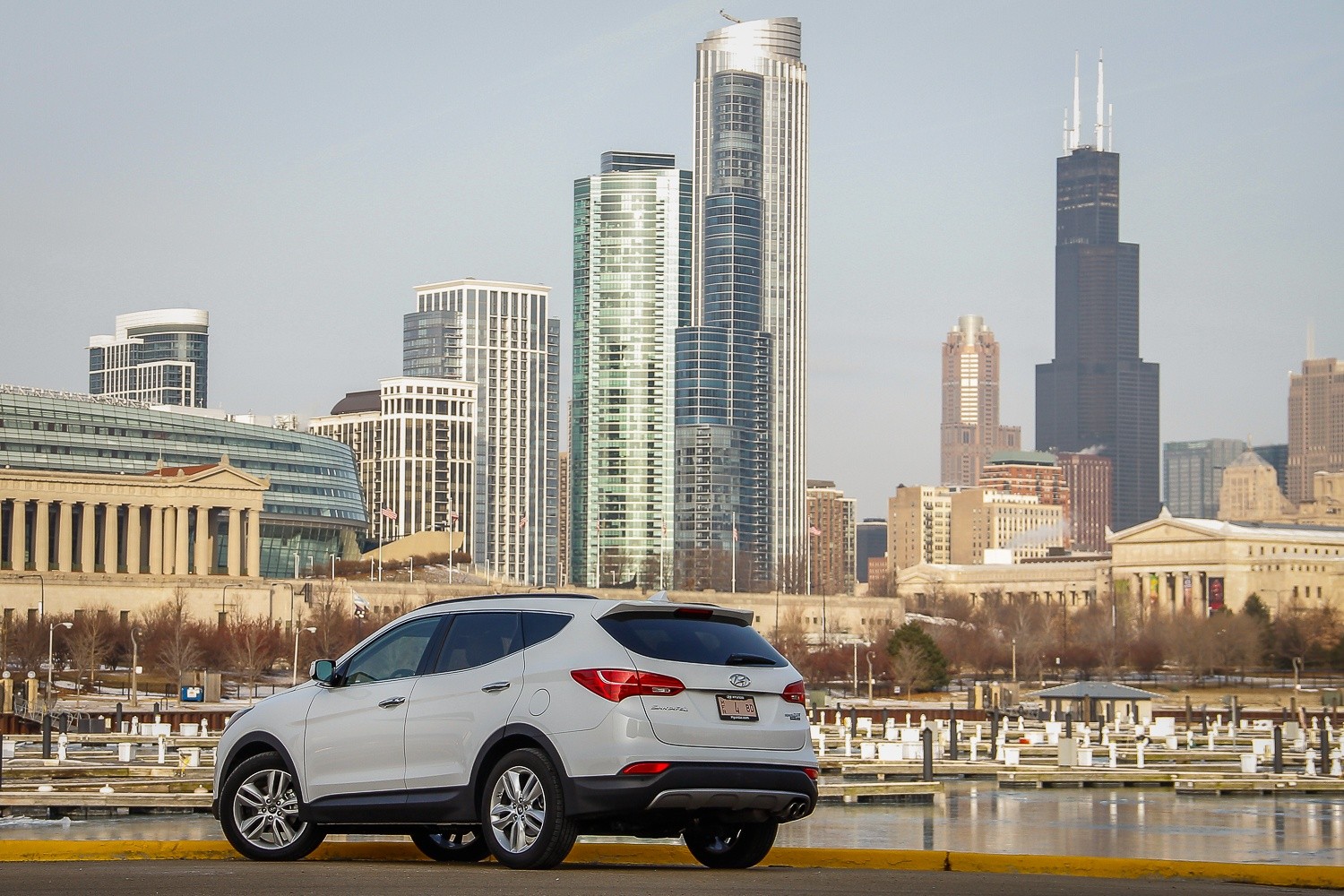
[210,588,822,871]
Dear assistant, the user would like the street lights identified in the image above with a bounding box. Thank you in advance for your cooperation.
[47,622,73,712]
[854,642,871,699]
[292,626,317,686]
[19,574,44,623]
[866,651,876,707]
[292,547,616,588]
[1011,637,1016,682]
[130,627,144,707]
[1292,657,1302,710]
[218,583,245,630]
